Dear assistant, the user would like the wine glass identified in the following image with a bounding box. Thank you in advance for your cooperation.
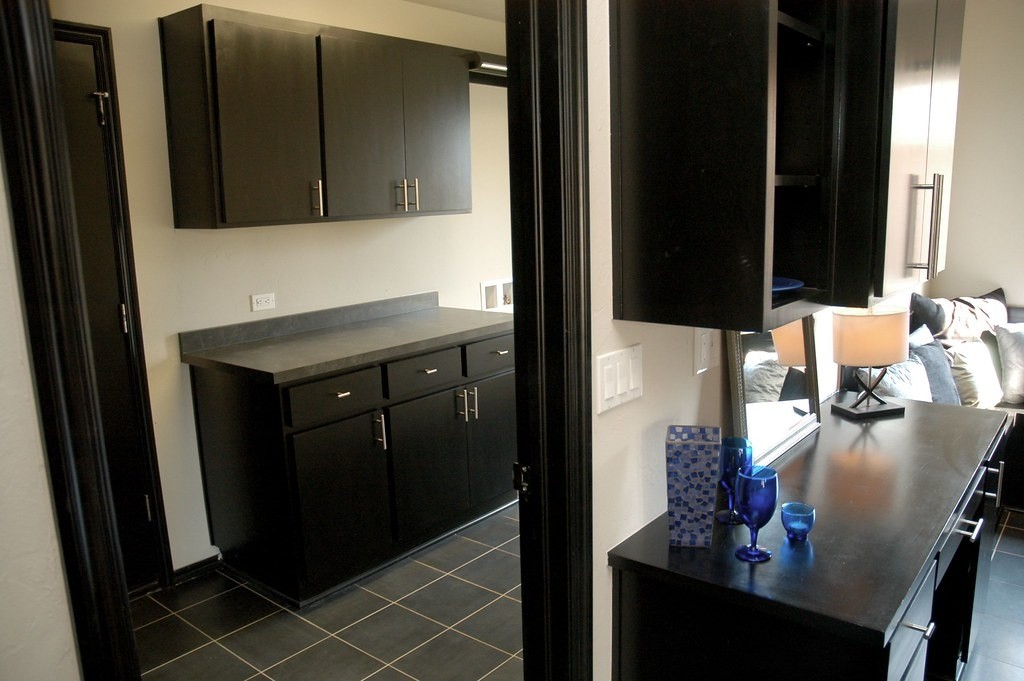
[731,464,780,564]
[715,435,754,526]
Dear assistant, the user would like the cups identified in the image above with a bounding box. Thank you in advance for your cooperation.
[779,503,816,543]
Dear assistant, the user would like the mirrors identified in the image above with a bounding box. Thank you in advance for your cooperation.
[724,313,823,472]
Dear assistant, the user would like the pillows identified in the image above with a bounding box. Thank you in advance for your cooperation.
[993,321,1024,405]
[946,331,1004,411]
[908,324,934,348]
[742,349,790,404]
[856,350,933,406]
[909,286,1009,349]
[778,368,807,402]
[911,339,961,407]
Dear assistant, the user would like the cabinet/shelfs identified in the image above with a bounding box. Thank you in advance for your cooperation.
[831,0,967,309]
[315,25,472,228]
[607,392,1014,681]
[189,363,399,608]
[607,0,848,332]
[379,330,516,561]
[157,4,325,230]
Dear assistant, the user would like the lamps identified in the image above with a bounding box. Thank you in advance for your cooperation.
[770,319,810,417]
[828,301,911,423]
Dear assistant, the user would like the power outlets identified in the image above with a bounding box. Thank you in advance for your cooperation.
[251,292,275,313]
